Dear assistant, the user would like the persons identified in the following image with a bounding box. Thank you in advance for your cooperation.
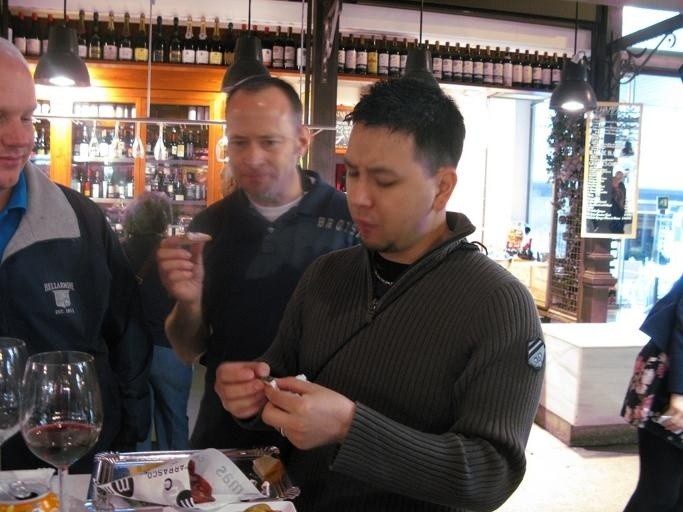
[152,76,363,450]
[116,190,191,454]
[618,275,682,511]
[212,74,546,512]
[0,37,154,476]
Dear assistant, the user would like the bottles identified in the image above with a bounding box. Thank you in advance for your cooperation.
[339,34,570,89]
[14,9,307,70]
[31,122,46,160]
[105,204,126,238]
[72,125,136,160]
[165,205,188,234]
[145,126,207,160]
[145,165,206,200]
[72,166,135,199]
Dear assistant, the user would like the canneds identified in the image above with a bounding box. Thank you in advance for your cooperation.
[0,481,60,512]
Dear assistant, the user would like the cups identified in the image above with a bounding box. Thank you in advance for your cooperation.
[33,101,136,121]
[187,107,209,121]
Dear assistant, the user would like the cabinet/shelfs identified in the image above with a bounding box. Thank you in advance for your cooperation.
[27,154,208,207]
[509,260,549,309]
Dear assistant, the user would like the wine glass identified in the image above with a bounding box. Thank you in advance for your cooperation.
[18,351,103,512]
[2,335,38,449]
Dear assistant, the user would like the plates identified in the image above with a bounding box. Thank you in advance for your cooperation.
[87,445,301,510]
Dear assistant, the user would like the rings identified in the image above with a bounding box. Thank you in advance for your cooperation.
[278,425,287,437]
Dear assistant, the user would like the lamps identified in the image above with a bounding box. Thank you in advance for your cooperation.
[549,2,599,115]
[404,0,434,76]
[36,0,90,90]
[217,2,273,92]
